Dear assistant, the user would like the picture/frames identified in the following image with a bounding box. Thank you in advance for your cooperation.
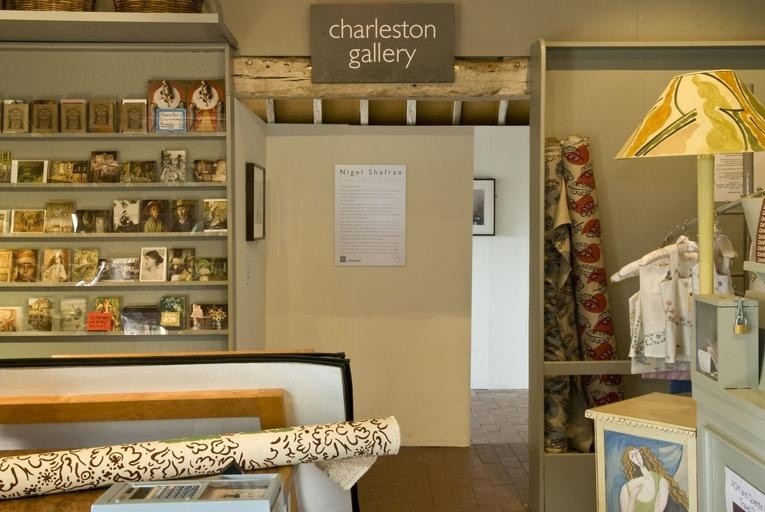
[471,177,496,236]
[246,162,265,242]
[0,91,149,134]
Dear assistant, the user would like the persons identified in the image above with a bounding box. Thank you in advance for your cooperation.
[44,252,66,281]
[119,209,130,225]
[169,197,197,233]
[141,250,165,280]
[12,248,36,281]
[142,198,167,233]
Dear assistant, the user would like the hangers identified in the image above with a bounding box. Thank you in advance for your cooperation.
[609,210,738,284]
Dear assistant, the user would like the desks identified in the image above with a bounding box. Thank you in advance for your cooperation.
[1,454,297,512]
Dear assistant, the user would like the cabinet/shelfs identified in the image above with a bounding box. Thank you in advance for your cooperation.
[1,43,233,362]
[690,293,765,512]
[584,390,698,512]
[530,38,764,512]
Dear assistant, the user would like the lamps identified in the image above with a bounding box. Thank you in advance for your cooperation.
[615,68,765,294]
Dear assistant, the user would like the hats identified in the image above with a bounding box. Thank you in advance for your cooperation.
[173,200,192,210]
[142,201,163,215]
